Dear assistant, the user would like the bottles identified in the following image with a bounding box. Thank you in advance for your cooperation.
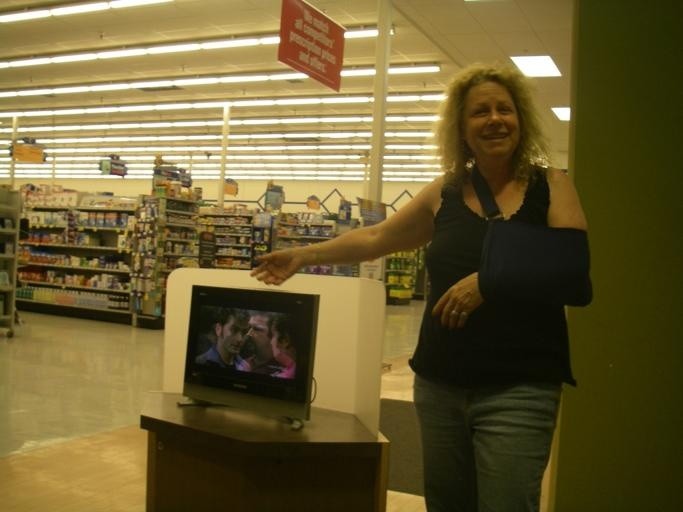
[387,259,413,300]
[17,285,129,311]
[128,204,154,314]
[166,241,184,254]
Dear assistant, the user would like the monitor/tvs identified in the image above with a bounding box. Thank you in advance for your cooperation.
[177,285,320,431]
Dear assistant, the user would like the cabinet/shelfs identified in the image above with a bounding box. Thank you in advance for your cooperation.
[0,183,430,337]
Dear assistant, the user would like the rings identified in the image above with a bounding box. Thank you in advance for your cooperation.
[450,311,468,321]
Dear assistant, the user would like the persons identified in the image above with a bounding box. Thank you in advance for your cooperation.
[196,307,312,378]
[250,64,593,512]
